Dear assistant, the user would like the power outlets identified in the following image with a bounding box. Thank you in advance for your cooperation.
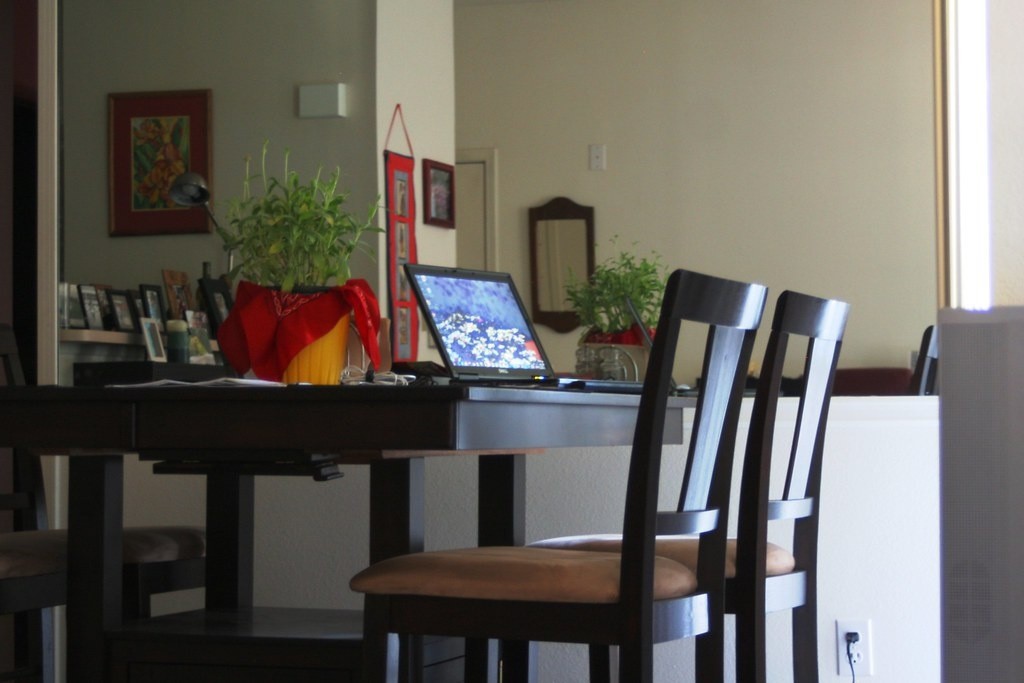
[834,618,871,678]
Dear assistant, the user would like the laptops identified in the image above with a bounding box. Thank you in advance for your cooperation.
[403,261,646,395]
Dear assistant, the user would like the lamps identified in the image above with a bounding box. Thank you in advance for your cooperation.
[168,169,236,300]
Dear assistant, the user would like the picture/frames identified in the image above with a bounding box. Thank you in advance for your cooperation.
[422,158,457,229]
[55,259,237,367]
[106,88,215,238]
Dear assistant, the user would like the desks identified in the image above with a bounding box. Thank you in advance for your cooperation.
[0,382,699,683]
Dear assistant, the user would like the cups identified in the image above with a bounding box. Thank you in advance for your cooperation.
[166,319,190,363]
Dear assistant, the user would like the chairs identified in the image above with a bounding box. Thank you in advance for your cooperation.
[0,320,207,683]
[501,289,853,683]
[907,322,939,396]
[348,268,770,683]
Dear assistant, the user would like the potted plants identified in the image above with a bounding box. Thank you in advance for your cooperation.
[576,234,674,383]
[216,139,394,384]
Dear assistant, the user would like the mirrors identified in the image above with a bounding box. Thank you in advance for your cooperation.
[528,197,596,334]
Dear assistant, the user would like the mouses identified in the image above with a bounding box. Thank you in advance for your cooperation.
[675,384,690,392]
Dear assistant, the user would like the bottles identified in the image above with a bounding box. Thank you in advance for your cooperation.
[576,347,598,380]
[599,348,625,381]
[194,261,213,313]
